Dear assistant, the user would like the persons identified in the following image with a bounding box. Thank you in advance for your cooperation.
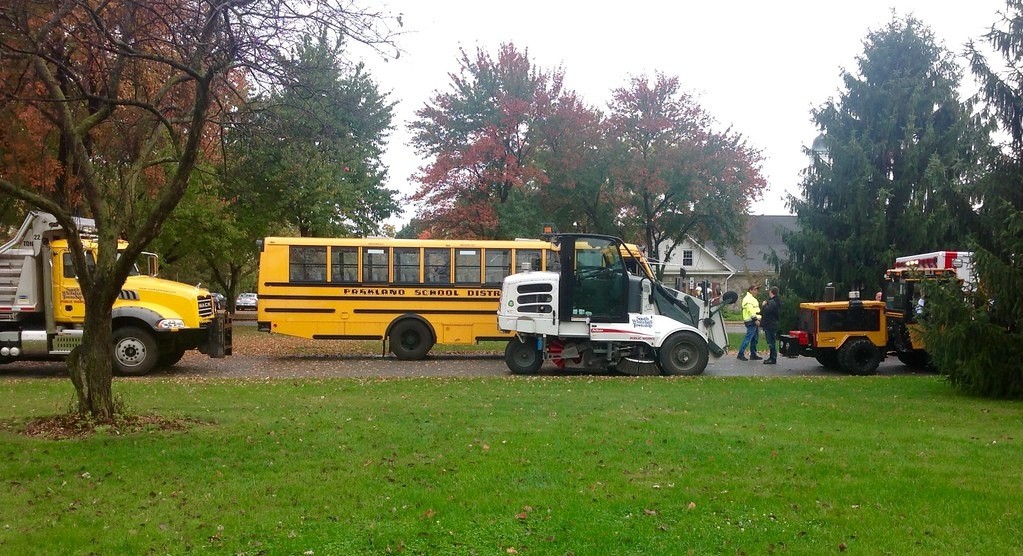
[736,285,778,364]
[875,292,882,301]
[695,283,712,300]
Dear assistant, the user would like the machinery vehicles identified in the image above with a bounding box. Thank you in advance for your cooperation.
[496,231,741,376]
[779,265,949,375]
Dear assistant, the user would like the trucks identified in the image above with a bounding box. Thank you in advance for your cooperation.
[0,213,233,375]
[895,252,983,310]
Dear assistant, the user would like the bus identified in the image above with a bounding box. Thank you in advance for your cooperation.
[257,233,660,360]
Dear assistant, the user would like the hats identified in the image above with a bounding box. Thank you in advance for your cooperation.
[748,286,759,290]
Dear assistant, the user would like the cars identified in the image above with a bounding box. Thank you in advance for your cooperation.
[237,292,257,311]
[209,293,227,308]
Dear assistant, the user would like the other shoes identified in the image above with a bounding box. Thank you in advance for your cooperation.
[750,356,762,360]
[736,355,748,361]
[763,358,776,364]
